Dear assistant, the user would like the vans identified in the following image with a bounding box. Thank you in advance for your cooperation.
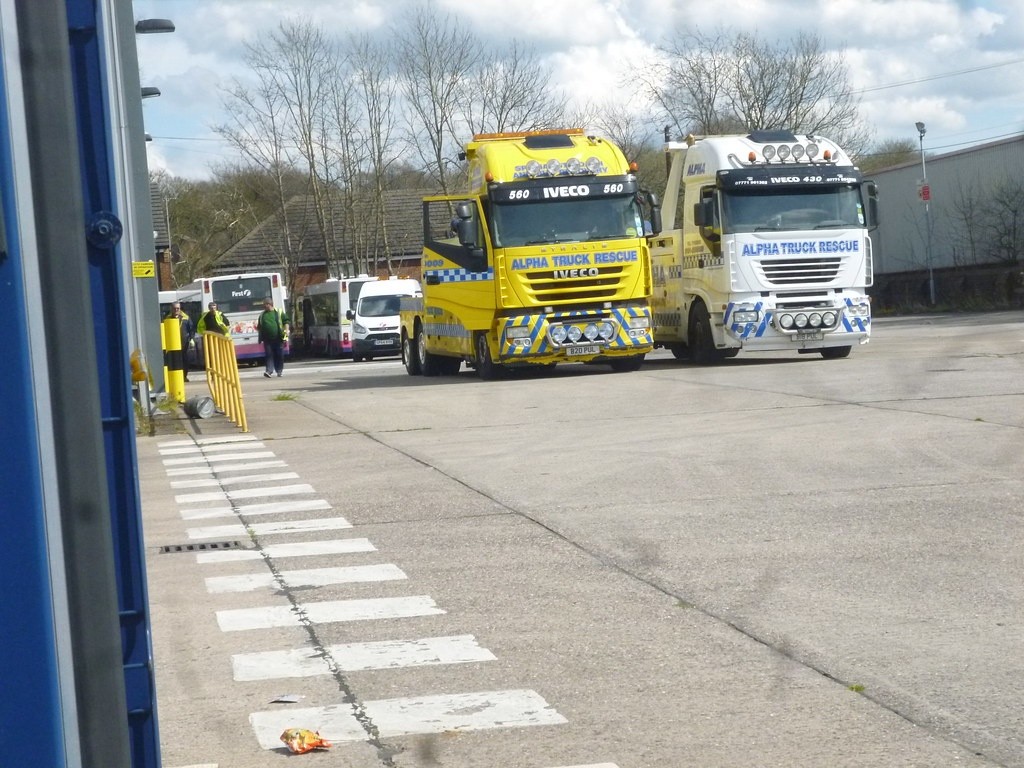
[345,274,424,363]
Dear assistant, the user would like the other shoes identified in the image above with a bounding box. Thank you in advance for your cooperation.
[184,377,189,382]
[263,371,272,378]
[277,373,283,377]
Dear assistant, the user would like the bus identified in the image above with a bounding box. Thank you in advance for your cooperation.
[298,274,381,358]
[178,273,291,367]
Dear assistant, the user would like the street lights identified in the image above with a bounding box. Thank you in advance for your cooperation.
[916,121,937,310]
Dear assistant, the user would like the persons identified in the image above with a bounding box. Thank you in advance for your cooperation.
[257,297,291,379]
[197,302,231,381]
[165,301,196,381]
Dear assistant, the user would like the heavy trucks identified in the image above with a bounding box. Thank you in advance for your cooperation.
[400,128,662,381]
[584,123,881,364]
